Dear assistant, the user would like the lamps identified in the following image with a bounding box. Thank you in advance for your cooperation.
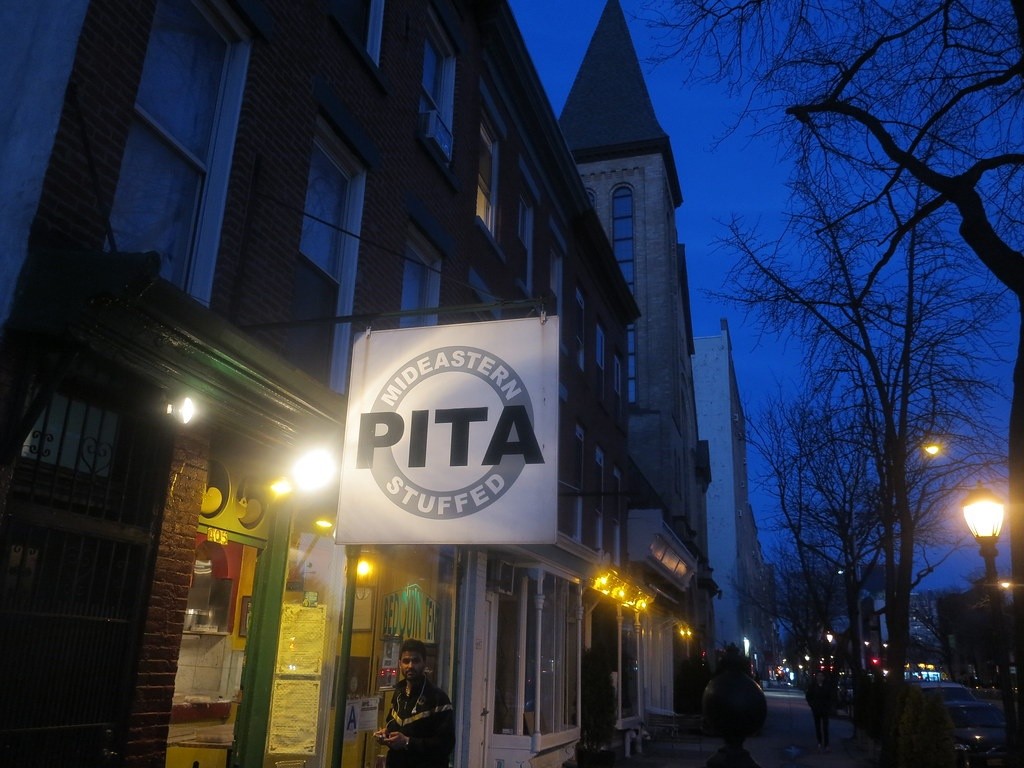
[159,392,193,424]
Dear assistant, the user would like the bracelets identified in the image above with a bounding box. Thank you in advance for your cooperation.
[406,737,409,745]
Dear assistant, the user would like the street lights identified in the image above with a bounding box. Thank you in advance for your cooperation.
[959,477,1021,768]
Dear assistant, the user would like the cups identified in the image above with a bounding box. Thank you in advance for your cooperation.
[184,608,215,632]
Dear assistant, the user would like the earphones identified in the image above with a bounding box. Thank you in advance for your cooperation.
[412,707,417,714]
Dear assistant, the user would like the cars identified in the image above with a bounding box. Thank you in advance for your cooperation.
[907,681,977,718]
[840,677,854,703]
[941,699,1011,768]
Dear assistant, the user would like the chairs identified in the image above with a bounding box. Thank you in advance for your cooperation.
[638,714,704,754]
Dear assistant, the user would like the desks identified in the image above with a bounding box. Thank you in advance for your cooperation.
[655,724,681,750]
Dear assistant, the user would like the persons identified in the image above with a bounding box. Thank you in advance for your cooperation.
[777,675,782,687]
[348,676,365,698]
[806,671,832,752]
[753,673,758,681]
[373,640,455,768]
